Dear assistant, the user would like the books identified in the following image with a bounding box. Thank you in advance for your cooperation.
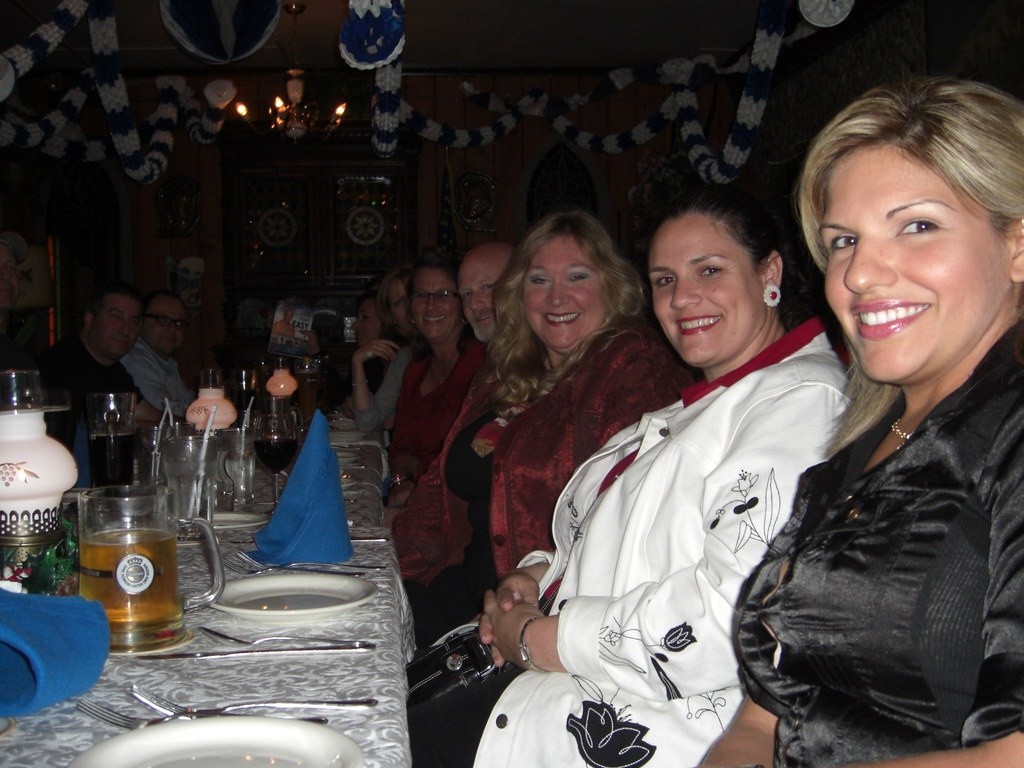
[267,301,314,359]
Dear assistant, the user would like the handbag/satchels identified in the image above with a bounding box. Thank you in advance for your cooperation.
[406,625,500,708]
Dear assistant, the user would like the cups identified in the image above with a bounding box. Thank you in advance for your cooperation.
[32,388,79,456]
[84,359,291,543]
[76,486,228,654]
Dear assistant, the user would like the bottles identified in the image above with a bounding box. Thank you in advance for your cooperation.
[0,368,79,538]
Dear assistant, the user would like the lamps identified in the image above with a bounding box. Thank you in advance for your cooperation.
[235,4,347,145]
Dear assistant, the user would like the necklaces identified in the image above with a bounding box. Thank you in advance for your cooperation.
[891,417,918,450]
[432,352,460,385]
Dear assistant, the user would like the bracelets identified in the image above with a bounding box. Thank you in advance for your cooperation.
[351,379,368,387]
[387,472,417,490]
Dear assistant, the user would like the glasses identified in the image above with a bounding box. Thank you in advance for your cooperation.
[410,290,458,302]
[143,313,190,332]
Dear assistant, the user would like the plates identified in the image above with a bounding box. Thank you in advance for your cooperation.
[67,714,365,768]
[210,512,271,530]
[210,572,379,623]
[321,417,374,491]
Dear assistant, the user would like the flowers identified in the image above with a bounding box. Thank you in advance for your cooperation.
[632,142,726,199]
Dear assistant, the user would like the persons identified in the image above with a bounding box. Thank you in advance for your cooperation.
[693,66,1024,768]
[405,181,853,767]
[378,210,706,659]
[36,284,201,491]
[299,283,411,431]
[0,231,38,408]
[347,239,543,595]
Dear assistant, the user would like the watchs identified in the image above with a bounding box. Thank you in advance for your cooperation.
[518,616,550,673]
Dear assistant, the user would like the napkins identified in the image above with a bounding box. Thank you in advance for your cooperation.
[245,407,354,565]
[0,588,111,717]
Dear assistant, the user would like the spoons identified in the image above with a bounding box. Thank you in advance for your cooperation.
[199,627,376,647]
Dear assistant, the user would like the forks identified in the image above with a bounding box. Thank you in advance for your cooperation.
[221,552,387,578]
[76,686,377,731]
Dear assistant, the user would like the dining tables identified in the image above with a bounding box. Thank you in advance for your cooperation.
[0,371,420,768]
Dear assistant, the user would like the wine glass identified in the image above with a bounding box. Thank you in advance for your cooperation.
[295,357,320,426]
[253,411,299,514]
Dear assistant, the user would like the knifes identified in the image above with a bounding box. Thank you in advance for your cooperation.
[135,640,375,659]
